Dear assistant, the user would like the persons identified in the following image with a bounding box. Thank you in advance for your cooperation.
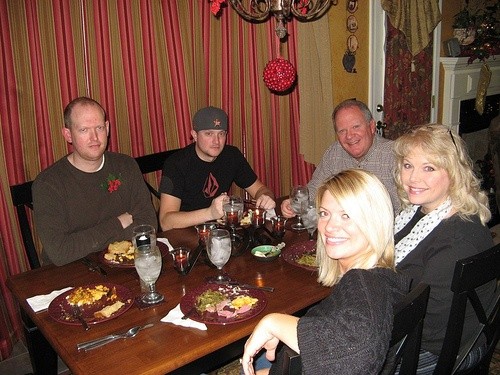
[240,123,500,375]
[159,107,275,232]
[31,97,157,267]
[281,100,404,218]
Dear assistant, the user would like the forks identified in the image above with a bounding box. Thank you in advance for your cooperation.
[71,305,89,330]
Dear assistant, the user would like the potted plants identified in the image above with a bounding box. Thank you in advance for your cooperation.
[451,7,476,45]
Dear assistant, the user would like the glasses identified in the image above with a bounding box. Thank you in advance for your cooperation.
[410,123,461,162]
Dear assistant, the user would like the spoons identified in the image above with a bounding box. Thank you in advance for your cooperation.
[85,326,141,351]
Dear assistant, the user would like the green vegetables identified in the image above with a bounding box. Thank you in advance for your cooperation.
[194,289,226,311]
[296,254,316,266]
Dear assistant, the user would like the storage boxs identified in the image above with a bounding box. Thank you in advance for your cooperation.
[443,38,461,57]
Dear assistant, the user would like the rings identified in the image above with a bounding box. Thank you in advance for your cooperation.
[240,359,251,365]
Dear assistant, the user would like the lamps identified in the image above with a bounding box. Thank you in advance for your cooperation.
[229,0,338,39]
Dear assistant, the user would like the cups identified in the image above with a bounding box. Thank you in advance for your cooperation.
[171,206,288,276]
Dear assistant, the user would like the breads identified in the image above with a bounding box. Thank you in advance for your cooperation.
[107,240,132,254]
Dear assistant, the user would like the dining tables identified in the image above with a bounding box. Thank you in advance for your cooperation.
[5,195,335,375]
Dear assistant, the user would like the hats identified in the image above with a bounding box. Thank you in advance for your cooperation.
[194,106,227,131]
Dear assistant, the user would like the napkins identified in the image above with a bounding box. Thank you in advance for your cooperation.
[26,287,73,312]
[139,235,175,252]
[160,303,208,331]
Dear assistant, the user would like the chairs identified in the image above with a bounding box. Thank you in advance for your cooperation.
[136,148,178,216]
[432,243,500,375]
[9,181,42,270]
[282,282,431,375]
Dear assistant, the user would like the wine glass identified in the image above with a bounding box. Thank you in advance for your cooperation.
[290,184,319,242]
[131,224,164,304]
[205,194,244,284]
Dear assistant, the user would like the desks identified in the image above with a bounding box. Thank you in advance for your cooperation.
[440,55,500,133]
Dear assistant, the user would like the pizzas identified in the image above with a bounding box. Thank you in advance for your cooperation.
[67,285,108,306]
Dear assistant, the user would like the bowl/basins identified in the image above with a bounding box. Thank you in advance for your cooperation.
[251,245,282,262]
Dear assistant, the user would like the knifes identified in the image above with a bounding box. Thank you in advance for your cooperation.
[77,323,154,350]
[83,258,108,275]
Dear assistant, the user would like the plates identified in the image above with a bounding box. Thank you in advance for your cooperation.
[99,240,169,268]
[47,282,134,326]
[181,282,269,325]
[282,239,321,271]
